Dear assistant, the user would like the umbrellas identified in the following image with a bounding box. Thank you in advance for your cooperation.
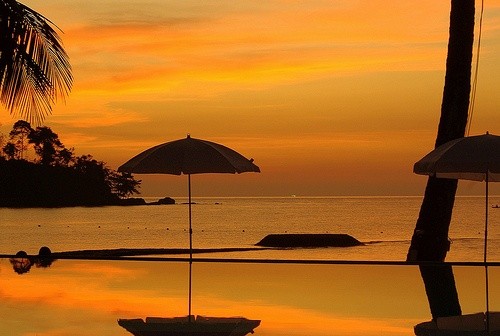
[412,133,500,262]
[118,262,260,336]
[414,265,500,335]
[118,138,260,235]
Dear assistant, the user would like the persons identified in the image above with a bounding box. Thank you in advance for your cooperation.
[39,246,51,255]
[17,251,26,256]
